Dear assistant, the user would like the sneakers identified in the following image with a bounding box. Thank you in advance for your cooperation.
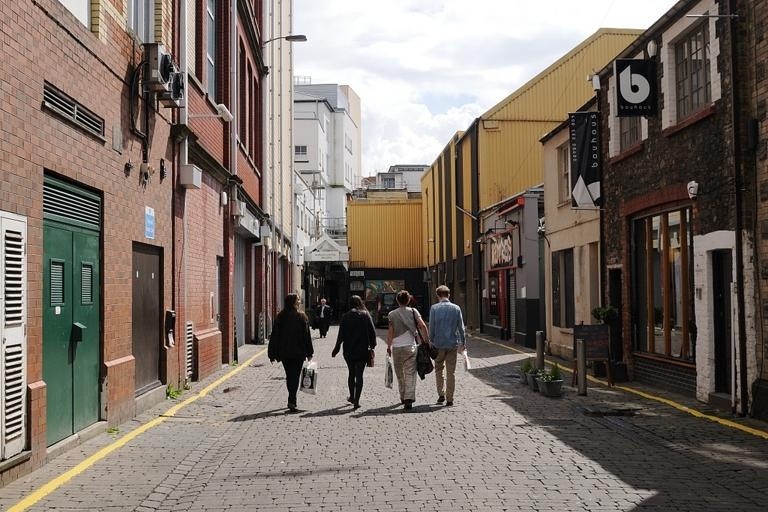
[287,402,301,414]
[437,395,446,403]
[445,401,454,406]
[346,396,362,411]
[400,396,415,411]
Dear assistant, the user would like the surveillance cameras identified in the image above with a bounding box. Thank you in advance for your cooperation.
[214,103,234,123]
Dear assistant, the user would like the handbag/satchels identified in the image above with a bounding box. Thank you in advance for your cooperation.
[299,361,318,396]
[366,349,376,367]
[421,348,434,375]
[461,347,472,373]
[383,351,394,389]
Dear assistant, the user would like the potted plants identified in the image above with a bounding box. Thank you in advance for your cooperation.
[515,354,565,397]
[590,302,621,328]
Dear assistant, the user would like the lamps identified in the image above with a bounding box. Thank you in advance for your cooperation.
[262,33,309,46]
[187,103,235,123]
[474,218,521,246]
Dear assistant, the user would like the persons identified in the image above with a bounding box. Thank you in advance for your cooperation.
[315,298,331,337]
[333,300,377,410]
[386,290,428,413]
[428,284,467,406]
[267,294,314,413]
